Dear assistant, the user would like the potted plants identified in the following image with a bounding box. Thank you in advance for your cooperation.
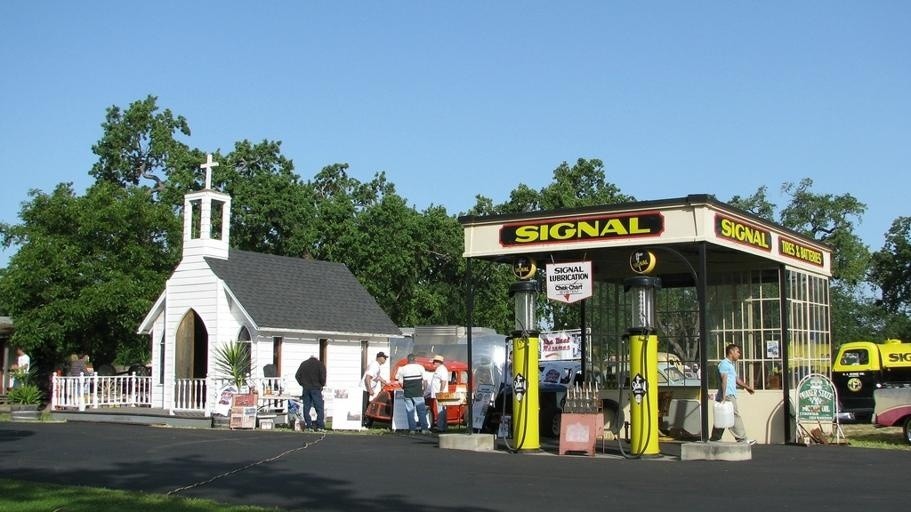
[6,365,42,422]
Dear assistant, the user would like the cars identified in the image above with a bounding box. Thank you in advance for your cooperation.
[364,356,473,424]
[496,359,607,438]
[604,352,705,389]
[871,388,911,444]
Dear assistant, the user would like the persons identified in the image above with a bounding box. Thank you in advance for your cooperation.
[398,354,432,435]
[710,344,755,447]
[70,352,90,405]
[295,354,327,433]
[430,355,449,434]
[362,352,391,431]
[13,346,30,389]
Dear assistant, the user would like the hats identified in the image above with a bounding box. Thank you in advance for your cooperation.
[433,355,444,363]
[377,352,389,358]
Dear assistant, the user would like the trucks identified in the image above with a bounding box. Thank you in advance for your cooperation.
[831,341,911,425]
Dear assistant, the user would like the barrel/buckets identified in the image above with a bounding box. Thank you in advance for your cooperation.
[713,399,735,429]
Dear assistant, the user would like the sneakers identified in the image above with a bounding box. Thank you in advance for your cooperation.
[409,430,432,435]
[748,439,757,446]
[307,427,324,432]
